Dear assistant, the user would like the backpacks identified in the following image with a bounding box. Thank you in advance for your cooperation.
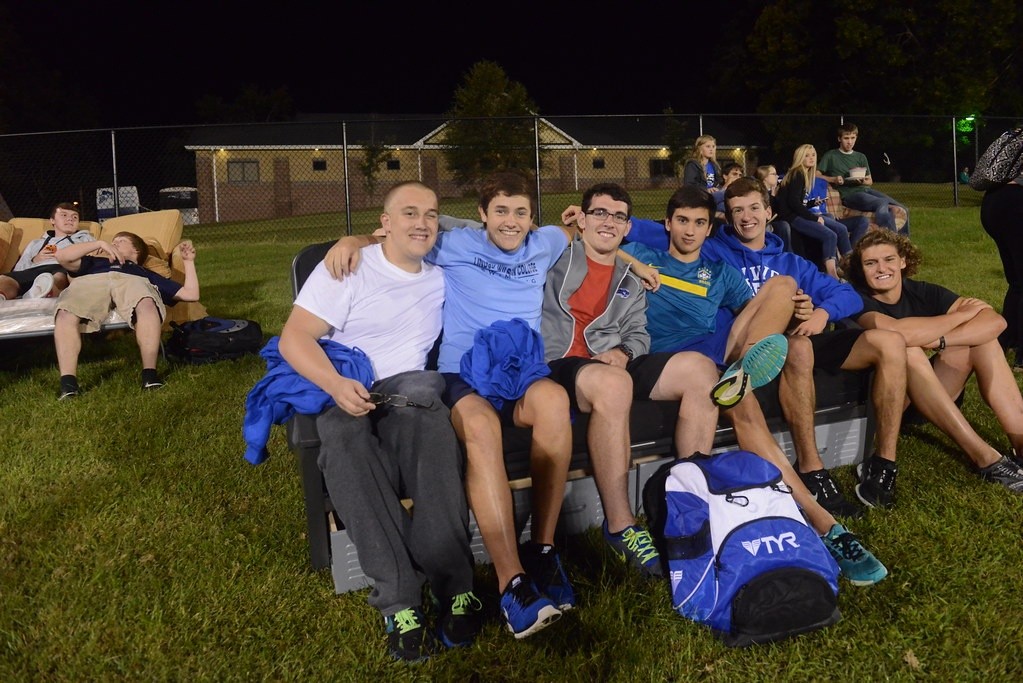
[969,127,1023,191]
[159,317,265,364]
[641,451,843,648]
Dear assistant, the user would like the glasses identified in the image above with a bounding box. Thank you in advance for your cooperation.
[586,209,628,221]
[768,173,777,175]
[367,393,435,410]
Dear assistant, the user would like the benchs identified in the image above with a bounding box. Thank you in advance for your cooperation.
[290,221,879,569]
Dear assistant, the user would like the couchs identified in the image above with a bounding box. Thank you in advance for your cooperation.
[0,208,191,366]
[826,187,907,276]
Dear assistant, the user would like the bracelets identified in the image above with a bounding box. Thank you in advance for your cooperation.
[932,336,945,352]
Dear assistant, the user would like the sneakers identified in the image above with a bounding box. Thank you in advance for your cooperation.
[792,459,857,520]
[855,454,898,508]
[528,544,576,611]
[602,518,664,578]
[963,451,1023,491]
[500,573,562,640]
[819,524,889,586]
[385,606,438,661]
[428,585,482,648]
[710,333,788,410]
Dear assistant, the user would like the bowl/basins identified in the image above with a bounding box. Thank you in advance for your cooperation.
[848,166,867,177]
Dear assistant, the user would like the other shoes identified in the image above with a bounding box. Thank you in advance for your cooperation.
[22,272,55,298]
[141,373,163,391]
[59,384,80,401]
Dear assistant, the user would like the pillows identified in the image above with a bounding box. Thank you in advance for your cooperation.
[161,298,208,332]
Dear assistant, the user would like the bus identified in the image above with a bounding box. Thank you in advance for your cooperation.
[161,187,199,228]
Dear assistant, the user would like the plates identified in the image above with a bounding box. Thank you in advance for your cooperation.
[845,175,869,180]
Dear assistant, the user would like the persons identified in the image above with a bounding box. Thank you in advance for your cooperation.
[839,229,1023,492]
[618,183,888,587]
[683,122,910,281]
[323,172,660,639]
[0,203,102,301]
[53,231,199,401]
[980,127,1023,373]
[561,177,907,519]
[959,166,970,184]
[372,182,719,576]
[277,180,482,662]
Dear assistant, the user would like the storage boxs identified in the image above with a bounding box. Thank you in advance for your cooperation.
[326,417,868,596]
